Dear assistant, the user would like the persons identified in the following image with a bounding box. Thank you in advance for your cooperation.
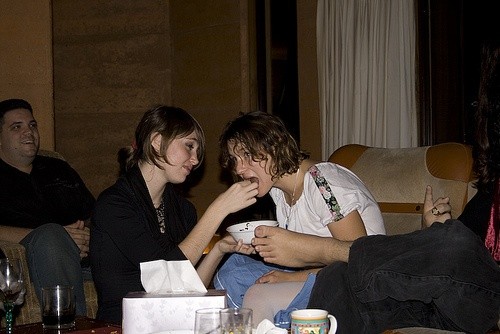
[251,44,500,334]
[0,99,104,322]
[211,108,385,334]
[88,105,258,325]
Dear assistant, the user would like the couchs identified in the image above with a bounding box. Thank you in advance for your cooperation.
[327,144,474,235]
[0,150,98,327]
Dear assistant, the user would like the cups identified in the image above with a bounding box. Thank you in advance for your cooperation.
[41,286,76,330]
[291,309,337,334]
[194,308,253,334]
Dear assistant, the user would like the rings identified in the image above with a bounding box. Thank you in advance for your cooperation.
[432,207,441,216]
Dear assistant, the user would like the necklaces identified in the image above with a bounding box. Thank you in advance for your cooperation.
[151,198,166,234]
[283,167,299,230]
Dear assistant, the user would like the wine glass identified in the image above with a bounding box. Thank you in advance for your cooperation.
[0,258,24,334]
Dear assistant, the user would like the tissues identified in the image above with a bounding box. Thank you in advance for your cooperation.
[120,258,228,334]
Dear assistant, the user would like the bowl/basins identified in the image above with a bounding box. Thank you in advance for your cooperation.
[226,221,279,244]
